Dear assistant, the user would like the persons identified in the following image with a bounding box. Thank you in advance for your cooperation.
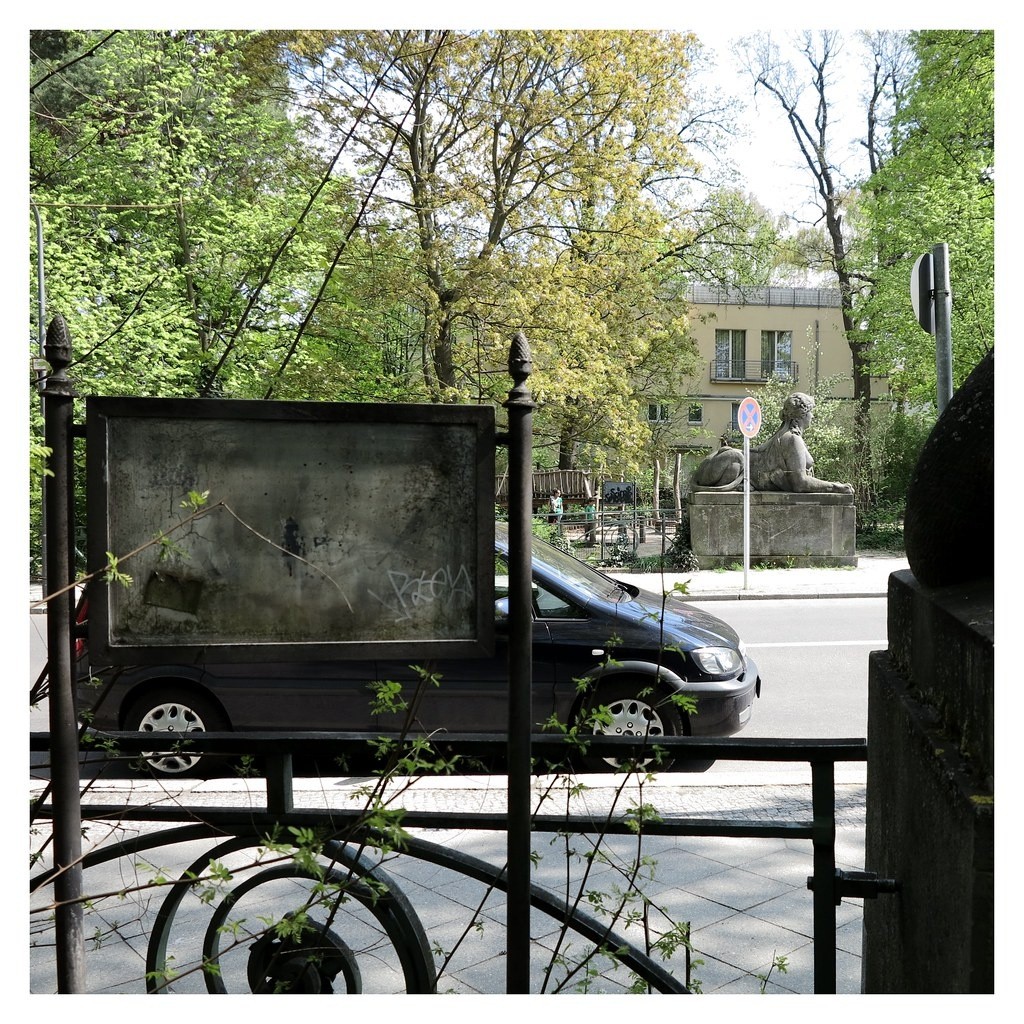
[584,498,596,542]
[546,488,563,526]
[689,393,853,493]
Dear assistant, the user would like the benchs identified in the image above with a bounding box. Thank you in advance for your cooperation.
[560,511,680,547]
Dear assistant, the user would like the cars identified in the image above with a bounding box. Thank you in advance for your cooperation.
[67,514,765,777]
